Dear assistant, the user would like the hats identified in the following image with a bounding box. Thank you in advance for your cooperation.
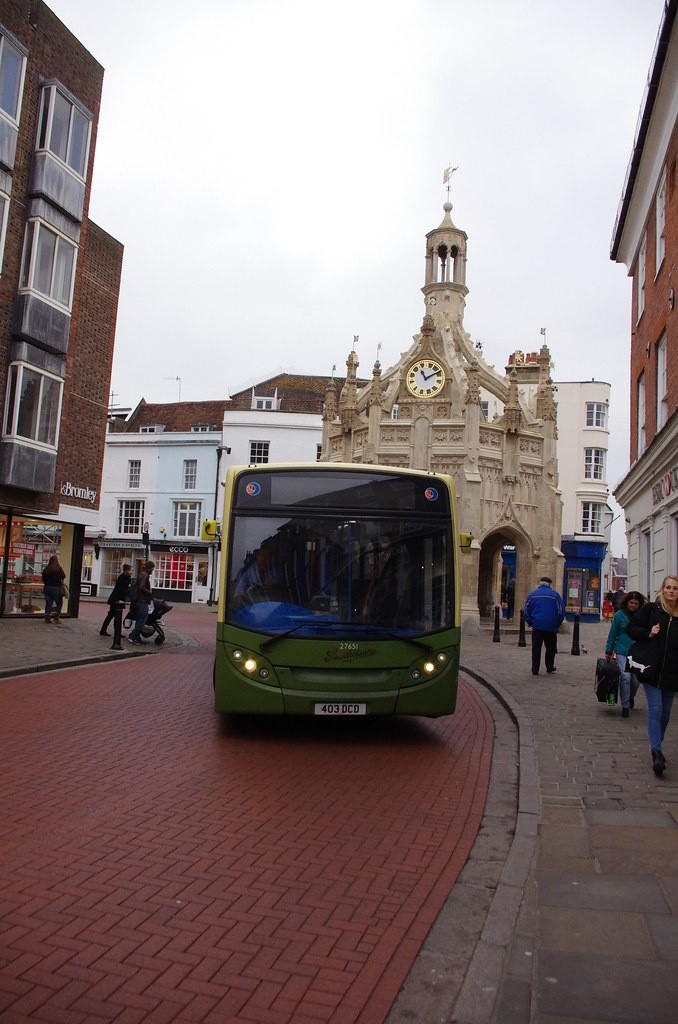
[541,577,552,584]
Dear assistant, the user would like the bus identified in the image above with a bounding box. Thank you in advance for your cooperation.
[202,460,477,719]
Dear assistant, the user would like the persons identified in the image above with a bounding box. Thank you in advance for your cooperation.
[42,556,66,623]
[605,590,645,717]
[128,561,155,646]
[501,577,516,621]
[612,587,624,613]
[234,537,289,596]
[310,598,329,615]
[99,564,133,650]
[626,576,678,775]
[606,590,614,600]
[524,577,565,675]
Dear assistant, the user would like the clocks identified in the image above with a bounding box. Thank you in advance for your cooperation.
[405,359,445,398]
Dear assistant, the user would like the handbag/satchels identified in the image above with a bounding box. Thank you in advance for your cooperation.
[61,584,66,597]
[501,601,508,609]
[624,602,659,677]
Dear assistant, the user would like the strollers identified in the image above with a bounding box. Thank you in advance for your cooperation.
[123,598,174,645]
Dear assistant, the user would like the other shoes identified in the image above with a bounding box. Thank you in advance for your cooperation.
[127,636,145,646]
[652,749,666,778]
[629,697,634,708]
[100,632,111,637]
[547,667,556,672]
[621,708,629,718]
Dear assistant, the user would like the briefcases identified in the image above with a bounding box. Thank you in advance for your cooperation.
[594,657,620,704]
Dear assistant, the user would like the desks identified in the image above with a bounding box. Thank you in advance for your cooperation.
[5,583,44,613]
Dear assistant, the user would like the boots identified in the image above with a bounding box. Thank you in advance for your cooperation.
[45,615,52,623]
[54,615,61,624]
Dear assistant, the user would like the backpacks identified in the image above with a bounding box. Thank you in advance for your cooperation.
[130,573,144,603]
